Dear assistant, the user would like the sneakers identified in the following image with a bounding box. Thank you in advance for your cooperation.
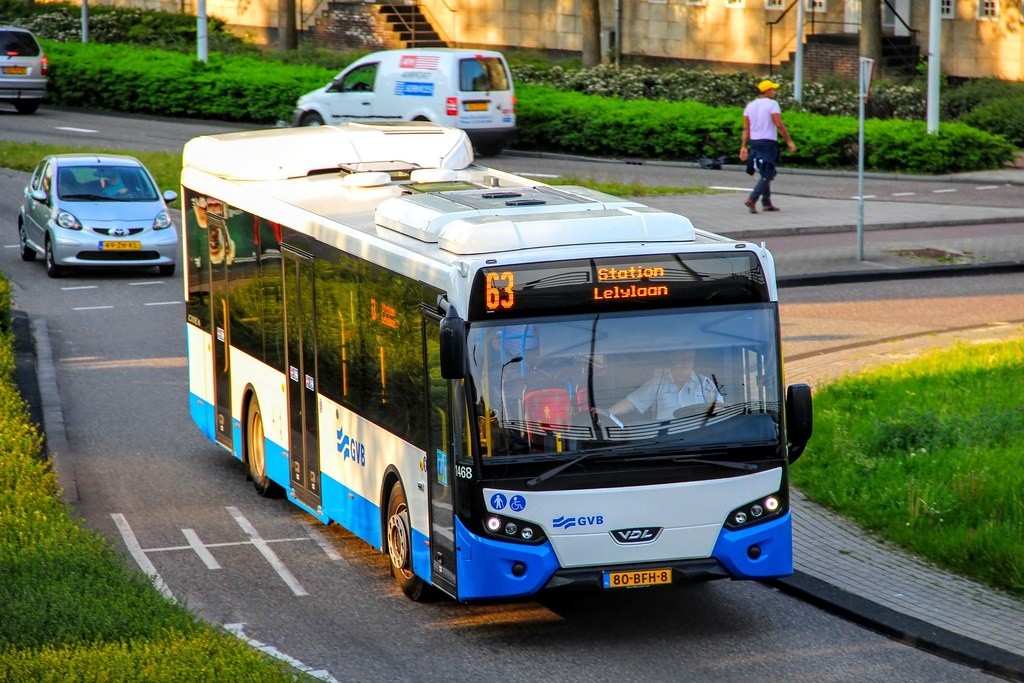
[745,198,758,214]
[763,204,779,211]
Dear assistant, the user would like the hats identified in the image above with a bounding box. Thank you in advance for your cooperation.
[758,80,779,92]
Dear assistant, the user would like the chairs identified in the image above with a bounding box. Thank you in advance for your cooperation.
[521,389,576,449]
[60,169,81,193]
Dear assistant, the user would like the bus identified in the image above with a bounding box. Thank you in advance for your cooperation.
[180,120,814,605]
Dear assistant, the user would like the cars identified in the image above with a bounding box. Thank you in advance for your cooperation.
[18,154,177,277]
[0,27,51,114]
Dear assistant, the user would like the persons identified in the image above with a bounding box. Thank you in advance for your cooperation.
[739,80,797,215]
[604,349,725,423]
[100,170,135,199]
[477,328,515,374]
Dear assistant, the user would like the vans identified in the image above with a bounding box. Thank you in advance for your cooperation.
[294,49,516,156]
